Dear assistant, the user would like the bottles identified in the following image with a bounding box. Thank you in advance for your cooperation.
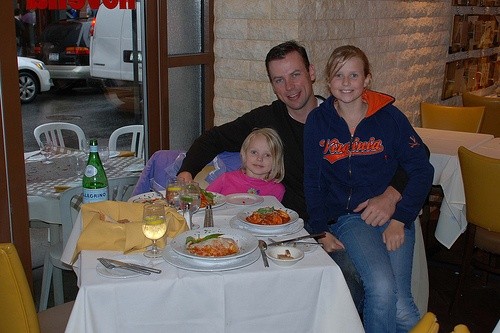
[81,140,109,204]
[203,205,214,228]
[183,205,192,230]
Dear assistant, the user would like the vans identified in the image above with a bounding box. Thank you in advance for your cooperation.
[89,2,143,104]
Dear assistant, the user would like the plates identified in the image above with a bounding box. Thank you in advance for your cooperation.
[225,192,264,205]
[96,262,143,279]
[161,244,261,272]
[171,226,259,261]
[239,205,298,231]
[127,190,226,214]
[230,216,306,237]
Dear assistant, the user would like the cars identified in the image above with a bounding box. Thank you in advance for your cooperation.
[17,57,54,104]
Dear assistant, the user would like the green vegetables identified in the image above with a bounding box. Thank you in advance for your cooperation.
[179,184,214,203]
[257,206,274,214]
[185,233,224,244]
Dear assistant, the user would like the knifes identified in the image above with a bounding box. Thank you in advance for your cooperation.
[268,232,326,245]
[258,240,270,268]
[96,258,162,274]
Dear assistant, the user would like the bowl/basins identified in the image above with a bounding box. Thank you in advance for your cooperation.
[265,246,304,265]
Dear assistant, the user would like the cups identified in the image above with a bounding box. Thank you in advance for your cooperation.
[166,176,189,212]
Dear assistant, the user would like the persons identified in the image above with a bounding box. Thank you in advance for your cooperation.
[302,45,435,333]
[205,127,286,203]
[14,8,38,57]
[175,39,430,333]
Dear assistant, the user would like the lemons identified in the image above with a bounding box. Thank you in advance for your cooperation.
[146,216,163,225]
[167,187,181,191]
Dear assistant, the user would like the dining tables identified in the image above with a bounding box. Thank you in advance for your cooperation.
[60,193,366,333]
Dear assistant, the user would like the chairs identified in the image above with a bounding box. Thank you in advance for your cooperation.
[34,122,87,151]
[108,125,144,159]
[420,101,486,133]
[458,146,500,272]
[39,176,140,311]
[0,242,75,333]
[462,92,500,137]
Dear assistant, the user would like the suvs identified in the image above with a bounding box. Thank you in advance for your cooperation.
[38,18,114,91]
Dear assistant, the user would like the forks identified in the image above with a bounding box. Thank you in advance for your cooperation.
[99,258,152,276]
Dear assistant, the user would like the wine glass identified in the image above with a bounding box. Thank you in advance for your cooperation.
[141,203,166,259]
[179,181,202,230]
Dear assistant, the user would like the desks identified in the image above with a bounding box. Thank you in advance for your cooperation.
[413,127,500,250]
[25,145,144,307]
